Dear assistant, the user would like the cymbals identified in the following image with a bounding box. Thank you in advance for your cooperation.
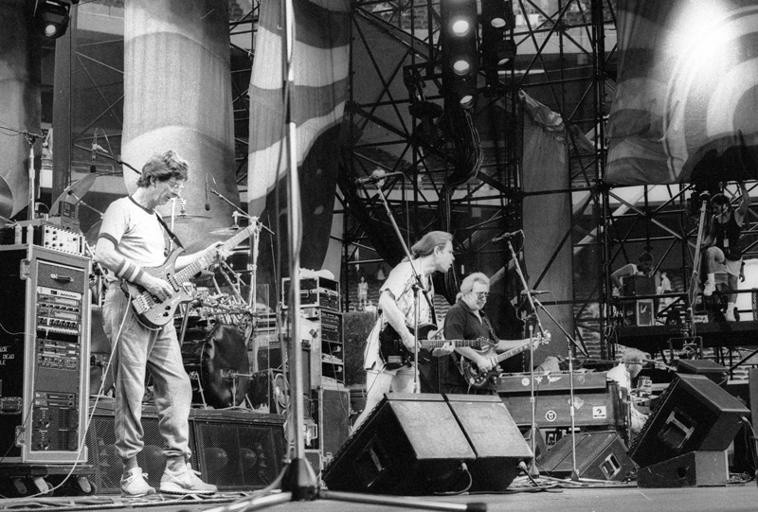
[49,173,96,216]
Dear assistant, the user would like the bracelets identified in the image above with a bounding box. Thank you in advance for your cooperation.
[618,287,621,290]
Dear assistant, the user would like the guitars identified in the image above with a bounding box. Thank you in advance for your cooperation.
[460,329,552,388]
[121,224,256,330]
[379,324,487,370]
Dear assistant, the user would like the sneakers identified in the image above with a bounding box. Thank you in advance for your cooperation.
[119,467,156,499]
[160,467,217,495]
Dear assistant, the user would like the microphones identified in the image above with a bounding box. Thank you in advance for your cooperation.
[491,230,521,244]
[354,171,402,188]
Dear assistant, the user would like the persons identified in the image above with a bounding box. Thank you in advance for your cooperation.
[367,300,376,311]
[703,177,751,323]
[93,151,235,495]
[444,272,539,492]
[661,272,673,311]
[354,230,456,492]
[607,351,650,435]
[356,276,368,311]
[610,252,654,326]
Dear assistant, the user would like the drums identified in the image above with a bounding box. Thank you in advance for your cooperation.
[174,287,215,322]
[177,325,249,410]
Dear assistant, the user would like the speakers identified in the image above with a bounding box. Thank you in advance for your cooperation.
[524,427,636,481]
[444,393,533,490]
[68,400,288,495]
[622,275,655,325]
[320,393,477,496]
[625,373,751,465]
[676,358,728,389]
